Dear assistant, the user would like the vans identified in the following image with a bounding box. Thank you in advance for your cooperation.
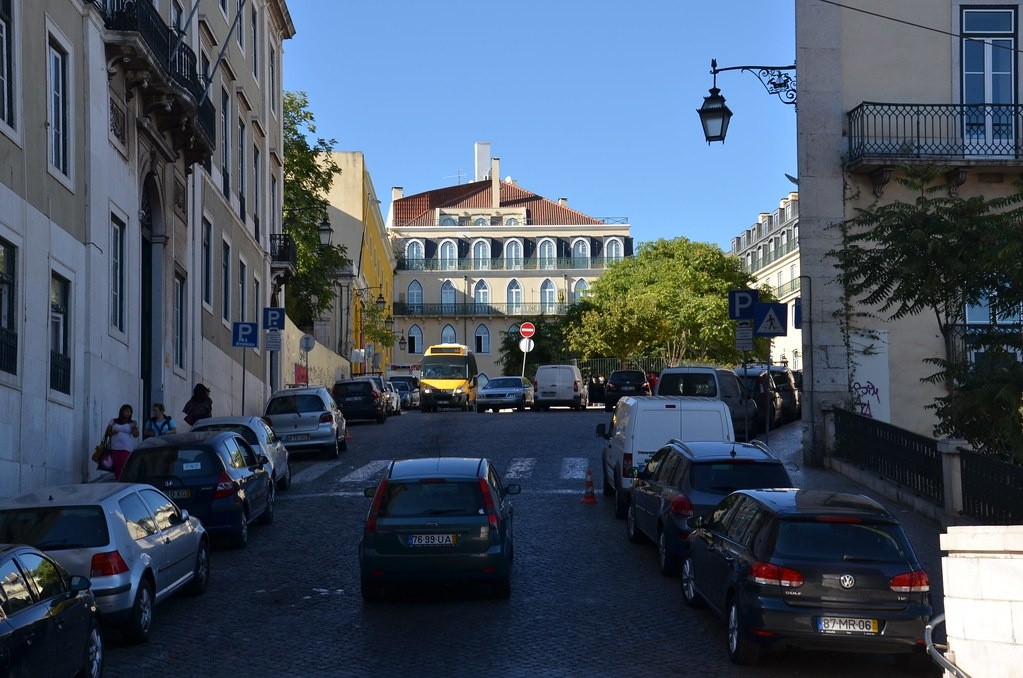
[535,365,588,411]
[596,396,735,518]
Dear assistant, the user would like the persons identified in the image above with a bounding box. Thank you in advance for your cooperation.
[103,404,140,480]
[583,370,659,406]
[145,403,177,436]
[185,383,213,426]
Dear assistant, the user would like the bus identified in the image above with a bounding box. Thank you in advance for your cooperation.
[419,343,478,413]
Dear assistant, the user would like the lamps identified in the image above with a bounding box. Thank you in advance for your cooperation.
[695,59,797,146]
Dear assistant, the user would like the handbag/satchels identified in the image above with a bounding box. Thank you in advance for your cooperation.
[184,398,196,424]
[93,432,115,471]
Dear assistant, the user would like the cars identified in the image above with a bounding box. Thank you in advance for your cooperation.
[117,431,275,547]
[0,483,210,642]
[358,456,520,601]
[261,386,346,459]
[655,362,802,442]
[678,488,933,664]
[589,369,660,412]
[186,416,291,500]
[332,375,419,424]
[626,439,793,578]
[0,543,104,678]
[476,375,534,412]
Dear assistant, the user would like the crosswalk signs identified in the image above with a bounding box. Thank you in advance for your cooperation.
[757,306,786,335]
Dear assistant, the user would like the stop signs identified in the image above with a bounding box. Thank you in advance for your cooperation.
[520,322,535,338]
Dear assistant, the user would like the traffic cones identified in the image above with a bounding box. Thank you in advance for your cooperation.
[579,469,600,503]
